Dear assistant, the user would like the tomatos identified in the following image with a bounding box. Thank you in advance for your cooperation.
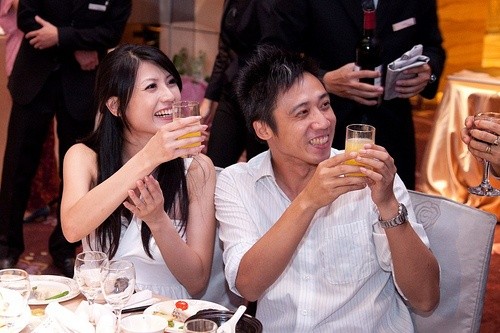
[175,301,188,310]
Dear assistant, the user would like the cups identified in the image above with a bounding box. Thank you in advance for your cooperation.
[119,314,169,333]
[344,124,376,177]
[182,319,218,333]
[171,100,203,158]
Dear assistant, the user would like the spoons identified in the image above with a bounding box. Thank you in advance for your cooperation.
[217,305,248,333]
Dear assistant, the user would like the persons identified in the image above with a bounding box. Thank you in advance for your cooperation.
[460,116,500,177]
[214,44,440,333]
[200,0,447,191]
[60,43,243,312]
[0,0,132,279]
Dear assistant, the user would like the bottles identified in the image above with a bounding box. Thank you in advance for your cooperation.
[353,5,382,107]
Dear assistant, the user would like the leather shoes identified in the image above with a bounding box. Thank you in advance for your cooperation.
[0,246,19,270]
[61,258,76,278]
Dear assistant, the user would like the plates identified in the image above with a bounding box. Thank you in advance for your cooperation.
[143,299,231,333]
[0,288,31,333]
[28,274,81,306]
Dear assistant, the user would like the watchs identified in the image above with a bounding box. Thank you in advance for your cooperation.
[378,202,408,228]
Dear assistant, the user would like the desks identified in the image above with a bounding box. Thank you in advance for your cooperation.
[419,76,500,221]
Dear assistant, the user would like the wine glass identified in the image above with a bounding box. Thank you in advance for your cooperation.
[0,269,31,333]
[73,250,109,333]
[100,259,136,333]
[468,111,500,198]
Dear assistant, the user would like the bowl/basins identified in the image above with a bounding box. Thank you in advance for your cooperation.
[78,269,119,304]
[186,308,263,333]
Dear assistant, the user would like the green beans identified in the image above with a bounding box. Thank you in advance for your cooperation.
[45,291,69,300]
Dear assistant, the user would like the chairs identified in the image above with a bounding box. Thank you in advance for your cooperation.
[408,190,497,333]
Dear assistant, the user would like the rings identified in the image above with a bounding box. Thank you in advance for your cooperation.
[486,145,491,153]
[493,135,498,145]
